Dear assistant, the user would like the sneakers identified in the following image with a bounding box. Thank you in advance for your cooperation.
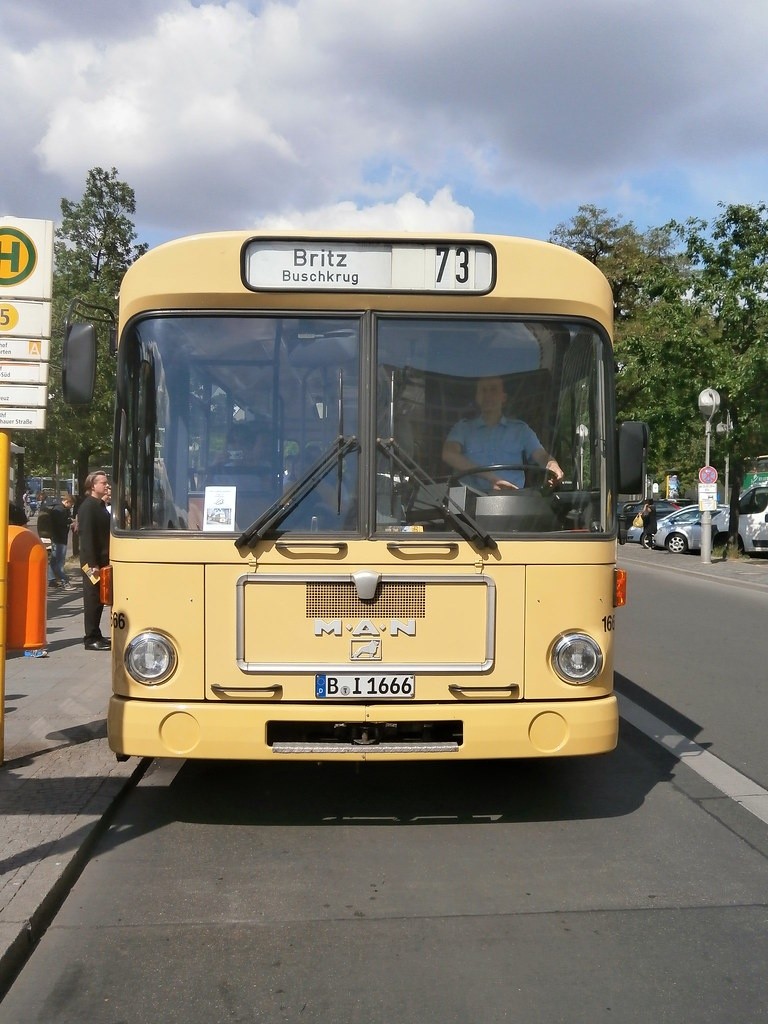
[65,584,77,590]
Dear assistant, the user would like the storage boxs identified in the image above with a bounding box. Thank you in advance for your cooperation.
[406,475,452,520]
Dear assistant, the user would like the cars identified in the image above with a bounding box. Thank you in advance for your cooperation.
[628,504,731,550]
[653,510,730,555]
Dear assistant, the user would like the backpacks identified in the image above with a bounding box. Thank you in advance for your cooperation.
[36,514,53,538]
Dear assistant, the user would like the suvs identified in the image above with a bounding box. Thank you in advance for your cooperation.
[614,498,679,543]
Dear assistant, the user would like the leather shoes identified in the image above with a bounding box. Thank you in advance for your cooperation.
[84,637,111,651]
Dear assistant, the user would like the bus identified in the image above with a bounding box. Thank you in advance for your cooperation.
[27,475,69,512]
[61,229,646,764]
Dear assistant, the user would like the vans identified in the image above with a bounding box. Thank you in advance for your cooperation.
[712,481,768,558]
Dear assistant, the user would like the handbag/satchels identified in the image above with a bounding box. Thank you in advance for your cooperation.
[633,511,644,528]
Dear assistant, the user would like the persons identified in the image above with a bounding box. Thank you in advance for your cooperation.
[638,498,657,550]
[440,365,565,531]
[201,422,349,518]
[22,470,132,657]
